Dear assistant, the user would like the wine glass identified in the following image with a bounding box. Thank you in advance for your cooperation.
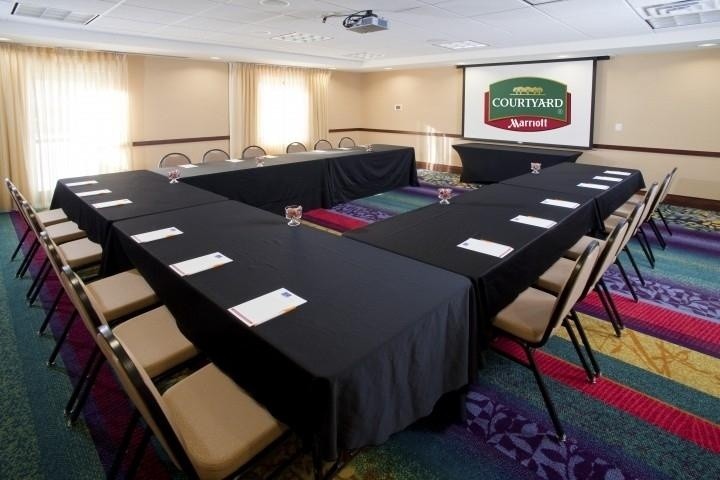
[530,162,541,175]
[256,156,264,166]
[168,170,179,184]
[285,204,302,226]
[438,187,452,205]
[365,144,373,152]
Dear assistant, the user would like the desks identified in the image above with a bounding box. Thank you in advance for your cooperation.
[49,171,470,480]
[151,143,419,216]
[453,143,583,185]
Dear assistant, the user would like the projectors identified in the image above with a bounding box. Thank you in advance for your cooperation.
[341,8,389,34]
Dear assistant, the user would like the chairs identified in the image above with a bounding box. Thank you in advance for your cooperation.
[41,230,157,416]
[11,187,87,299]
[159,136,356,169]
[5,179,68,278]
[22,201,103,334]
[97,326,306,478]
[61,265,208,480]
